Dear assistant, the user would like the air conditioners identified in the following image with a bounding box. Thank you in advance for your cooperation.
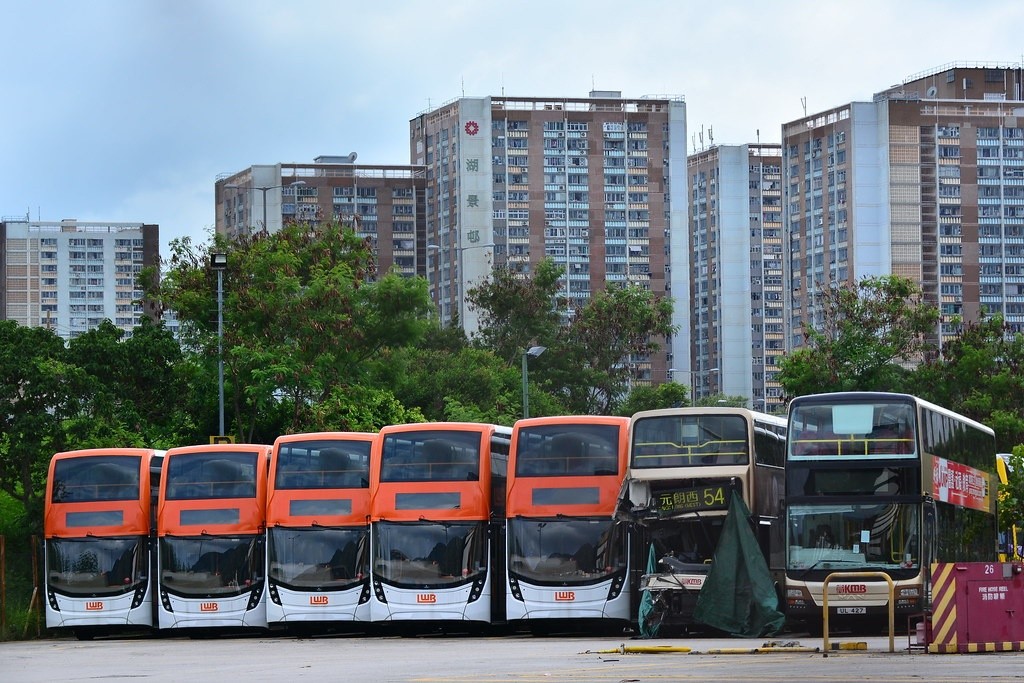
[629,363,636,368]
[955,296,963,302]
[755,280,761,286]
[545,220,551,225]
[405,189,412,194]
[942,129,952,137]
[522,167,528,173]
[559,131,564,137]
[558,185,565,190]
[580,132,586,137]
[393,245,399,250]
[698,182,702,187]
[581,231,588,238]
[603,133,609,138]
[578,213,583,219]
[557,168,565,173]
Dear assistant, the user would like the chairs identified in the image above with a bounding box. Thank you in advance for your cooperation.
[641,441,734,464]
[796,431,829,454]
[874,432,899,454]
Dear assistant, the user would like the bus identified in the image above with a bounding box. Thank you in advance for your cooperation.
[370,421,613,637]
[44,448,255,639]
[611,406,818,637]
[264,432,509,638]
[996,454,1024,562]
[505,415,631,637]
[783,392,998,636]
[157,444,367,638]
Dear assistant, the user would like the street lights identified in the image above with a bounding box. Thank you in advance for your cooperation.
[210,251,227,435]
[521,346,546,419]
